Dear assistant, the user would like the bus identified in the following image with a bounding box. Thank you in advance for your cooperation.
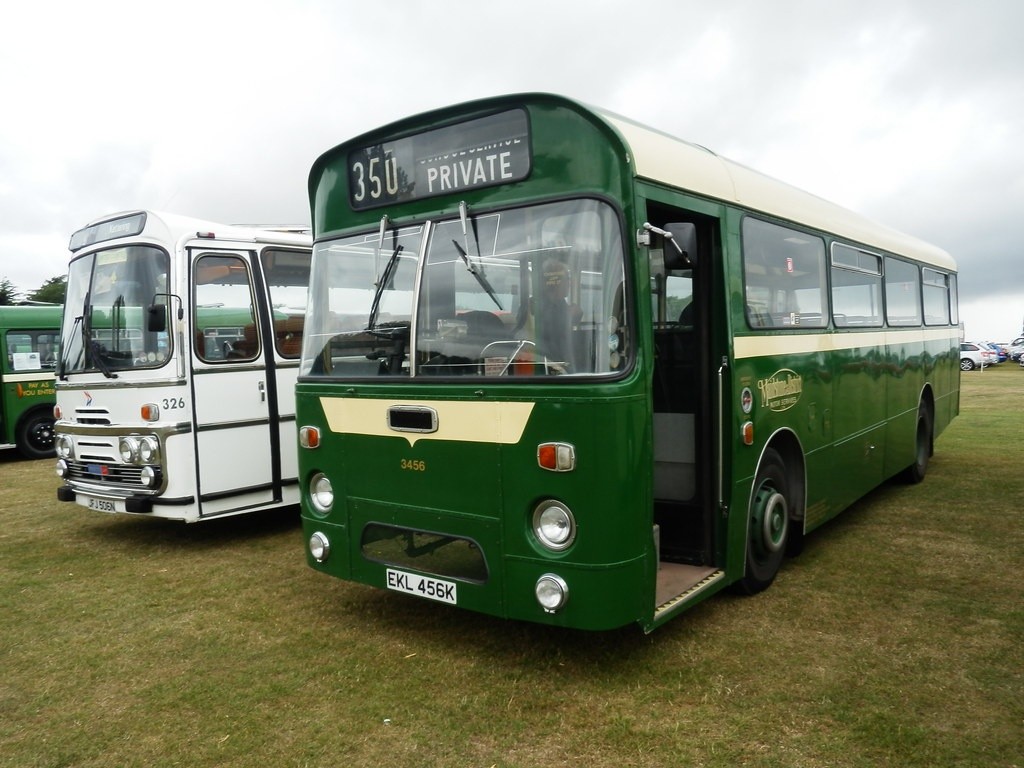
[0,300,289,460]
[54,208,671,524]
[295,92,961,636]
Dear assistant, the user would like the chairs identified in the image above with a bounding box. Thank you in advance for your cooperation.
[750,312,945,329]
[242,311,392,359]
[567,319,694,412]
[39,340,56,365]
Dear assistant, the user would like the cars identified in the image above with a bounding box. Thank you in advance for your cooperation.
[959,338,1024,371]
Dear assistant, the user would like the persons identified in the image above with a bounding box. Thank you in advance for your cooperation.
[518,259,583,375]
